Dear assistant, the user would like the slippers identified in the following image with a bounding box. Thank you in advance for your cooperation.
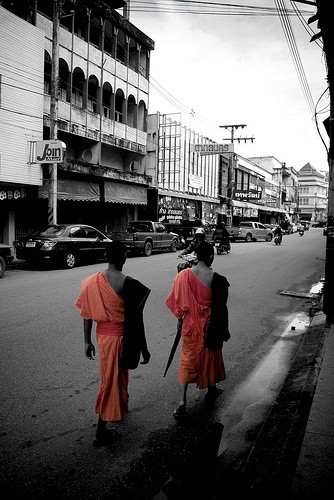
[205,387,223,398]
[173,409,188,417]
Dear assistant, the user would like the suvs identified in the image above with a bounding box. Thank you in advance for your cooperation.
[0,244,14,279]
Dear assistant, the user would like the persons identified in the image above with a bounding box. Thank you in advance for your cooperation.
[179,228,206,257]
[273,222,305,239]
[165,242,231,418]
[213,221,230,253]
[74,240,151,444]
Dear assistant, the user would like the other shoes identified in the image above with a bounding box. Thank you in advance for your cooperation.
[95,427,122,441]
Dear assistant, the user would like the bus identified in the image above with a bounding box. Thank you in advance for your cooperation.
[229,221,273,243]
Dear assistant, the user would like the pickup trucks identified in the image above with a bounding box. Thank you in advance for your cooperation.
[112,220,179,256]
[170,219,212,249]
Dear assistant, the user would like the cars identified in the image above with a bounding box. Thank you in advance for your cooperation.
[268,221,309,235]
[16,224,112,269]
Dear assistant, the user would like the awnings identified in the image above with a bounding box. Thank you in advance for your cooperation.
[229,200,287,213]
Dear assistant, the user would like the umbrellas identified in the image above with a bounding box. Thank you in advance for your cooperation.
[163,329,181,377]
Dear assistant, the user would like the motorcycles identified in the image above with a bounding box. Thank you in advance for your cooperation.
[215,235,231,255]
[177,250,213,275]
[274,233,283,245]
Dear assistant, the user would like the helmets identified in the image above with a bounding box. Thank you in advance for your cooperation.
[221,222,225,227]
[195,228,205,234]
